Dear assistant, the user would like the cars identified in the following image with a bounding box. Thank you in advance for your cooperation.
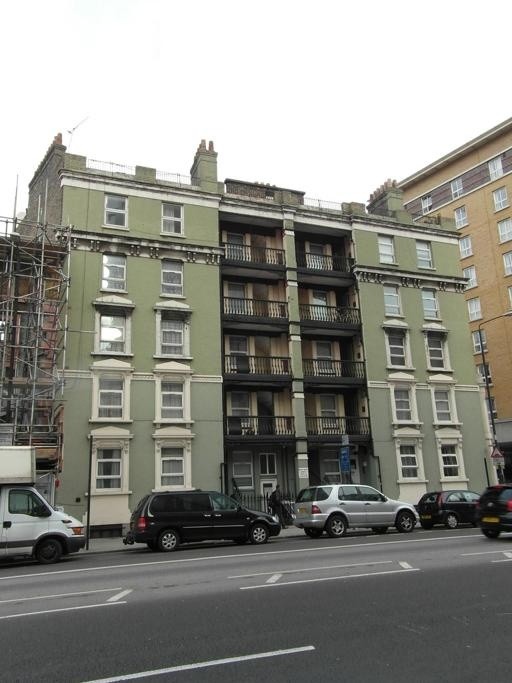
[475,484,512,537]
[292,483,421,537]
[418,489,483,528]
[129,488,281,551]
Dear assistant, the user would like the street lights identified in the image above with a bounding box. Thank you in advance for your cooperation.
[478,310,512,484]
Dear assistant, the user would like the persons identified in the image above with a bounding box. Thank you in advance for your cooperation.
[267,483,289,530]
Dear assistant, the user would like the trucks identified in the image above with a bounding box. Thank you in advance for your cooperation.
[0,444,85,564]
